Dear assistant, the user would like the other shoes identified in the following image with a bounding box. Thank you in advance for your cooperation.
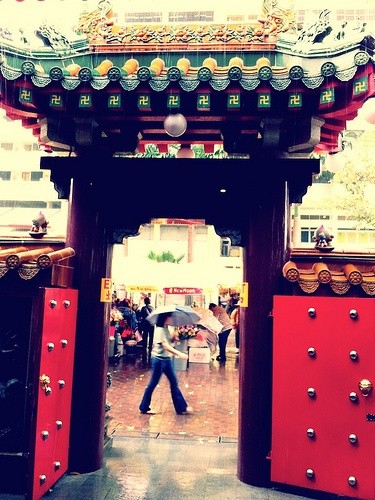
[139,408,155,413]
[216,355,226,361]
[182,409,193,415]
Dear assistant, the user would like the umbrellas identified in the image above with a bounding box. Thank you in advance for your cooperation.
[145,305,202,341]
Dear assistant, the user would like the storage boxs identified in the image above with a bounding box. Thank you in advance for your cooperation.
[174,339,211,370]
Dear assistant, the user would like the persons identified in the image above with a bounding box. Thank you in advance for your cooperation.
[217,292,240,314]
[107,289,155,366]
[229,301,240,354]
[136,312,193,414]
[209,302,232,361]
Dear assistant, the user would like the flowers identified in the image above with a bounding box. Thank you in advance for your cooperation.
[177,324,200,341]
